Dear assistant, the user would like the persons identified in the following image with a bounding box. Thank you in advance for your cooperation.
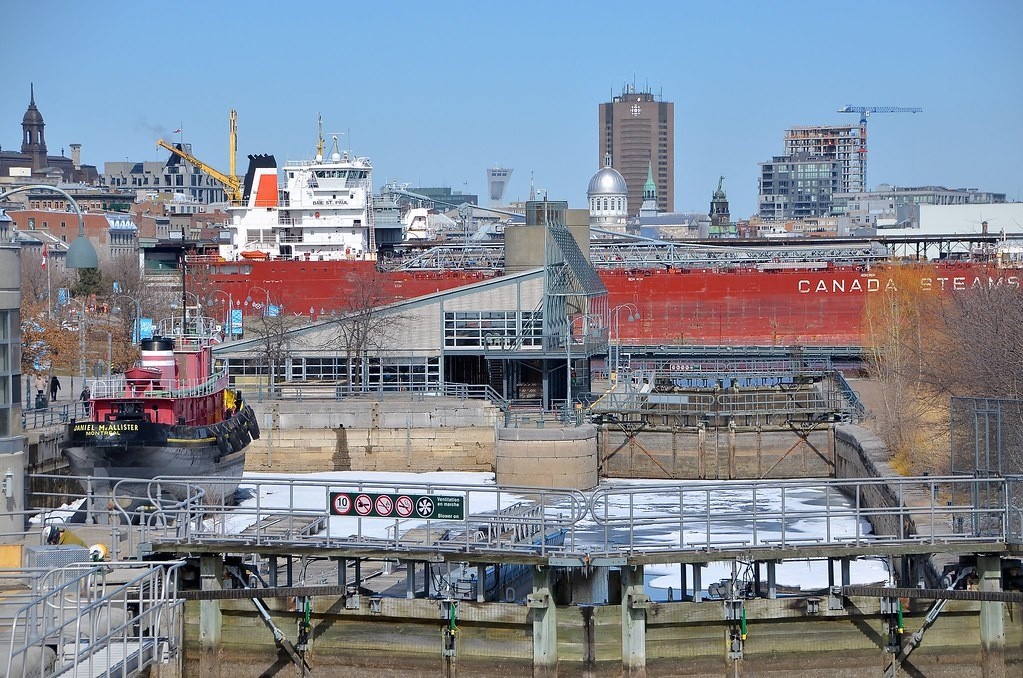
[42,526,90,550]
[34,373,46,398]
[50,375,61,402]
[79,386,91,416]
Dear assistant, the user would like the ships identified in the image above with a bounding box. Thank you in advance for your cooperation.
[57,224,260,526]
[178,116,1022,388]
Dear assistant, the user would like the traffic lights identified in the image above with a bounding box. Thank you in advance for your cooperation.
[571,371,576,385]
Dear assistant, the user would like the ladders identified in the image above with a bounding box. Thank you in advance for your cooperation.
[879,566,978,678]
[229,574,315,678]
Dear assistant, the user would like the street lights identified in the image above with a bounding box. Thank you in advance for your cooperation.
[169,289,203,345]
[53,297,90,393]
[608,304,634,390]
[615,302,641,391]
[567,316,599,423]
[111,296,142,367]
[246,287,272,318]
[208,290,234,343]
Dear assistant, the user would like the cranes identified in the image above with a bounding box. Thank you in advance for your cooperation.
[837,105,923,194]
[154,108,241,208]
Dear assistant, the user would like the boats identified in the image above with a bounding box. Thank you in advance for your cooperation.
[240,250,268,259]
[123,366,162,393]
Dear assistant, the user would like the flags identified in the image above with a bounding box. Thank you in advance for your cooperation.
[41,244,47,272]
[174,128,181,133]
